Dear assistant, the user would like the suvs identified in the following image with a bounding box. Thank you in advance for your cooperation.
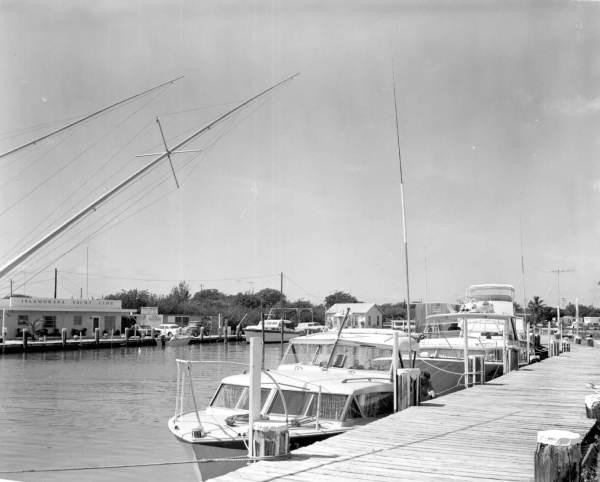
[184,320,210,337]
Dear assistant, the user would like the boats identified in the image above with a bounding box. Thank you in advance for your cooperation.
[423,285,530,396]
[243,321,323,344]
[167,329,423,481]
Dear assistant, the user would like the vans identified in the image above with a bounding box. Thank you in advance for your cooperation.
[158,324,180,337]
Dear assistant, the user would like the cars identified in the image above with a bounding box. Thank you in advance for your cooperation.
[132,324,158,337]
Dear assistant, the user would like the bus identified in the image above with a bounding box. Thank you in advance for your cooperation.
[392,320,415,330]
